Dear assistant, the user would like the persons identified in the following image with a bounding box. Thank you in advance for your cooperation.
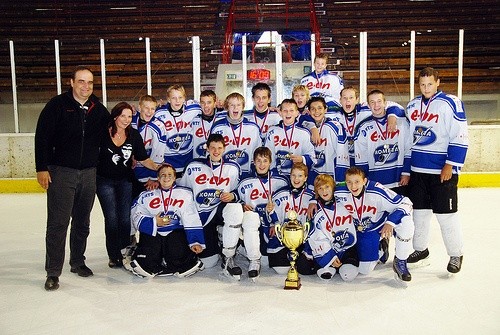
[95,83,288,282]
[35,66,111,291]
[265,66,468,289]
[300,53,344,108]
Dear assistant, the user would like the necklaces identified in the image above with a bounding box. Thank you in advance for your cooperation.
[117,133,124,139]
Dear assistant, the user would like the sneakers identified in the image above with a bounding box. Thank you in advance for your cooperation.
[405,248,430,269]
[446,254,464,276]
[220,255,242,282]
[380,239,390,264]
[248,258,261,286]
[392,257,411,287]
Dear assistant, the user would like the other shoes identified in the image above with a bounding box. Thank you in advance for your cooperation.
[105,258,125,269]
[70,263,94,277]
[45,276,59,291]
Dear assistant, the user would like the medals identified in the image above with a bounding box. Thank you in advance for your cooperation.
[350,140,353,144]
[285,154,290,158]
[163,215,168,222]
[216,190,222,198]
[358,226,363,231]
[384,144,389,148]
[176,136,180,141]
[287,210,298,221]
[418,128,422,133]
[203,143,207,149]
[331,232,336,237]
[236,152,240,156]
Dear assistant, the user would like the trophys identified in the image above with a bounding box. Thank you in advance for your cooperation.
[275,219,310,290]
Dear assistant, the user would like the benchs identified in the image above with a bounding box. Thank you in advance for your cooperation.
[0,0,500,105]
